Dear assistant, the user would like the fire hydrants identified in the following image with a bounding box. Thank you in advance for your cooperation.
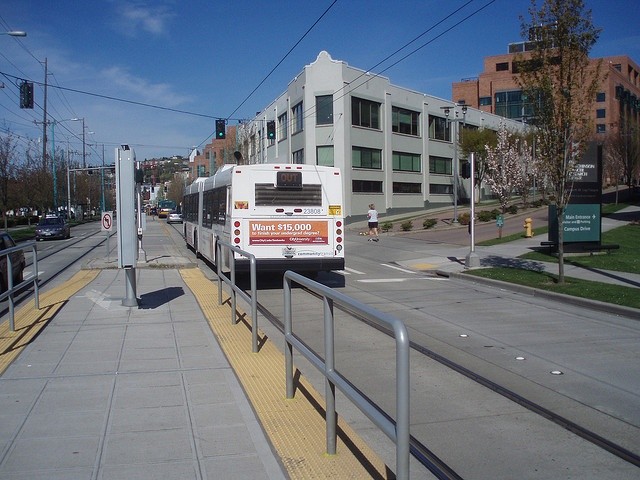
[523,218,533,237]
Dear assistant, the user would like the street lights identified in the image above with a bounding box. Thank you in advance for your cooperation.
[61,132,95,206]
[1,31,26,36]
[51,118,78,207]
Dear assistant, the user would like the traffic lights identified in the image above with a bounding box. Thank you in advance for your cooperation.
[164,187,167,192]
[267,122,275,139]
[216,120,225,139]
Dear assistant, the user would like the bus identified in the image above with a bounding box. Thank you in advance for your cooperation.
[158,201,178,218]
[182,163,345,278]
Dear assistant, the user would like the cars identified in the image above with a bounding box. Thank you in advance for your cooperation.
[35,216,70,241]
[167,210,184,224]
[0,232,25,295]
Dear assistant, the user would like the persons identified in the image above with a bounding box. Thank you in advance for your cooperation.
[366,203,379,242]
